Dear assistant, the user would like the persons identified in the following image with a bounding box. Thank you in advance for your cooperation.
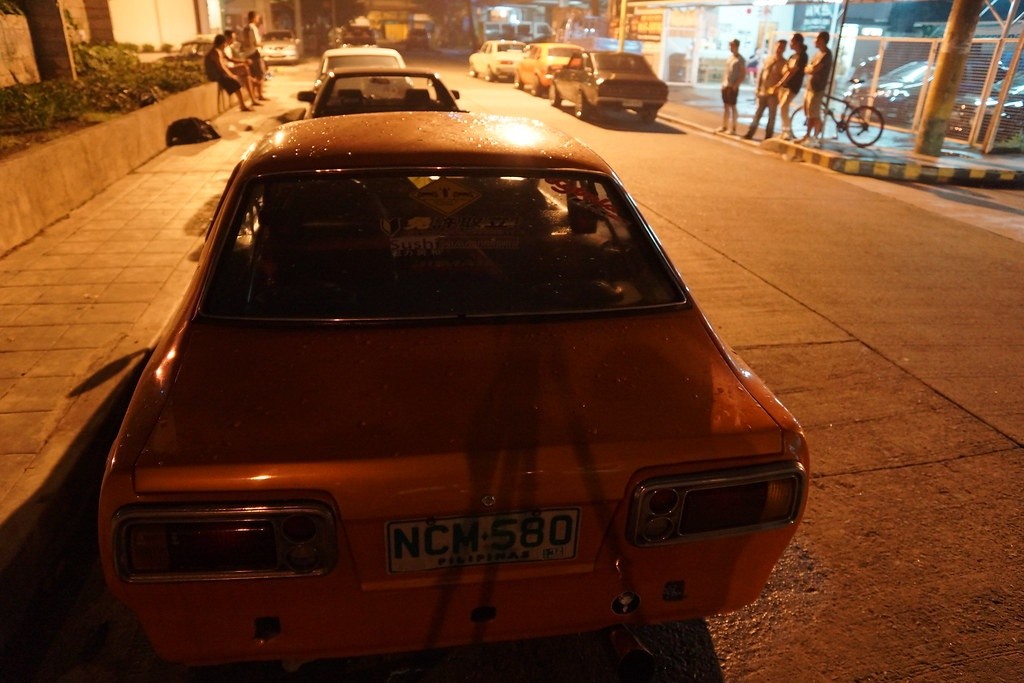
[794,32,833,148]
[205,11,271,111]
[768,34,808,140]
[713,39,745,136]
[742,39,789,140]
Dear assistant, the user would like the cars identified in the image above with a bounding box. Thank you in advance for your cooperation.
[177,34,217,55]
[259,29,301,64]
[297,66,461,118]
[467,38,527,82]
[512,42,585,97]
[946,69,1024,139]
[549,50,670,124]
[92,111,814,661]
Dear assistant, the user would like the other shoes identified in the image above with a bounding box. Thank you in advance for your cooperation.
[782,128,792,140]
[740,134,752,140]
[252,103,260,106]
[801,138,820,148]
[726,131,736,134]
[253,80,261,87]
[241,106,250,111]
[794,135,811,144]
[259,97,266,100]
[718,127,726,132]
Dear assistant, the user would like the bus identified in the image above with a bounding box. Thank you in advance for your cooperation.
[340,17,378,47]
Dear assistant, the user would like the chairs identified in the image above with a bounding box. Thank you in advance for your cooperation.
[404,88,429,100]
[337,88,362,104]
[273,173,390,241]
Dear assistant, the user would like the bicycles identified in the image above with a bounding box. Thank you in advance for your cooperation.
[789,76,886,148]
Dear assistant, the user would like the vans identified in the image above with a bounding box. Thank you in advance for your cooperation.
[317,47,414,104]
[405,28,429,51]
[843,60,1004,124]
[478,17,551,39]
